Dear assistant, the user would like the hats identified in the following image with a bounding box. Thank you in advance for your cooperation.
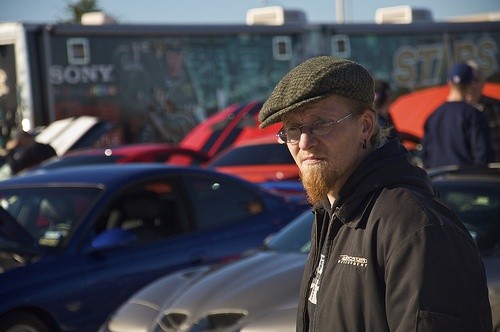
[448,62,479,86]
[257,56,374,130]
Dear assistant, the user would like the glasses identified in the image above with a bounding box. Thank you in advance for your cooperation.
[276,111,359,145]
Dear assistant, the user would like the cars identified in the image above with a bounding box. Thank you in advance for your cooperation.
[18,144,210,176]
[103,163,500,331]
[0,164,313,332]
[209,123,301,184]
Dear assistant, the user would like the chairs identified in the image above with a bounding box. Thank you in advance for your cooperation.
[88,190,180,251]
[37,193,78,249]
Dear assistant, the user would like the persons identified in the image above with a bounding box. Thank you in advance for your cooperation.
[369,78,404,146]
[462,59,500,164]
[257,56,494,332]
[417,62,492,175]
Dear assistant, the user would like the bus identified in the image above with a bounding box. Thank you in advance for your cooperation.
[1,7,500,147]
